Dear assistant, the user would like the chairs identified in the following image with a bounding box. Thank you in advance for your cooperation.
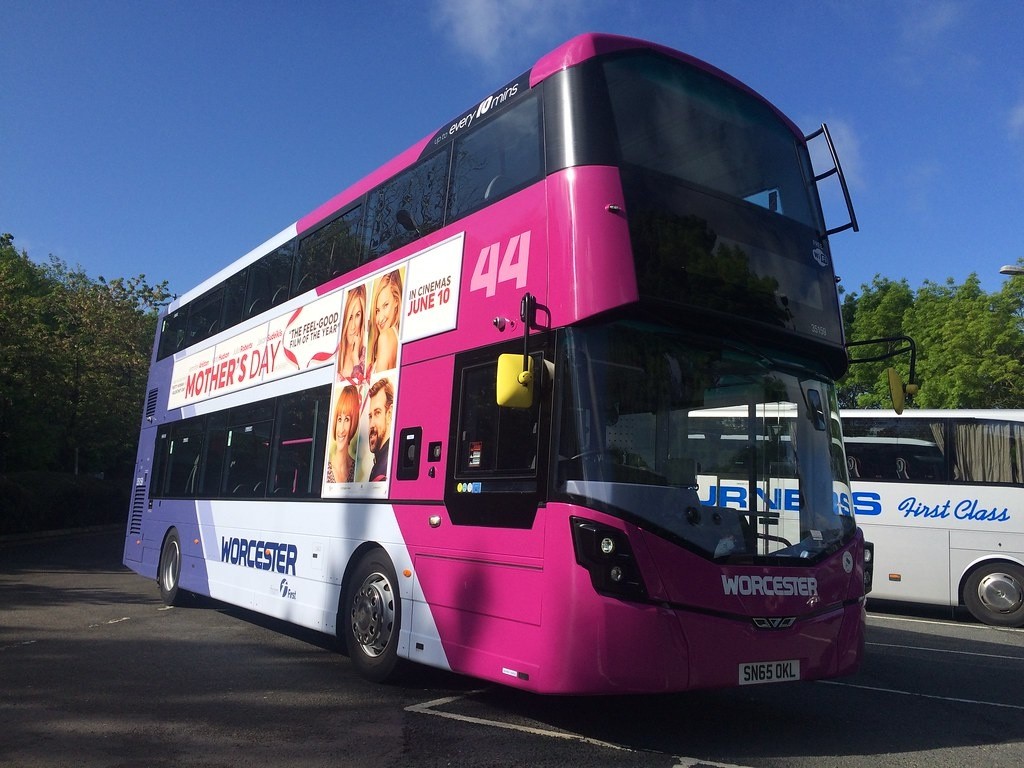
[895,458,913,478]
[177,271,324,345]
[485,175,523,200]
[847,456,864,478]
[234,481,292,497]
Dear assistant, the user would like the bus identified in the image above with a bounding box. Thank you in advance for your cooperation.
[123,33,919,705]
[686,403,1024,630]
